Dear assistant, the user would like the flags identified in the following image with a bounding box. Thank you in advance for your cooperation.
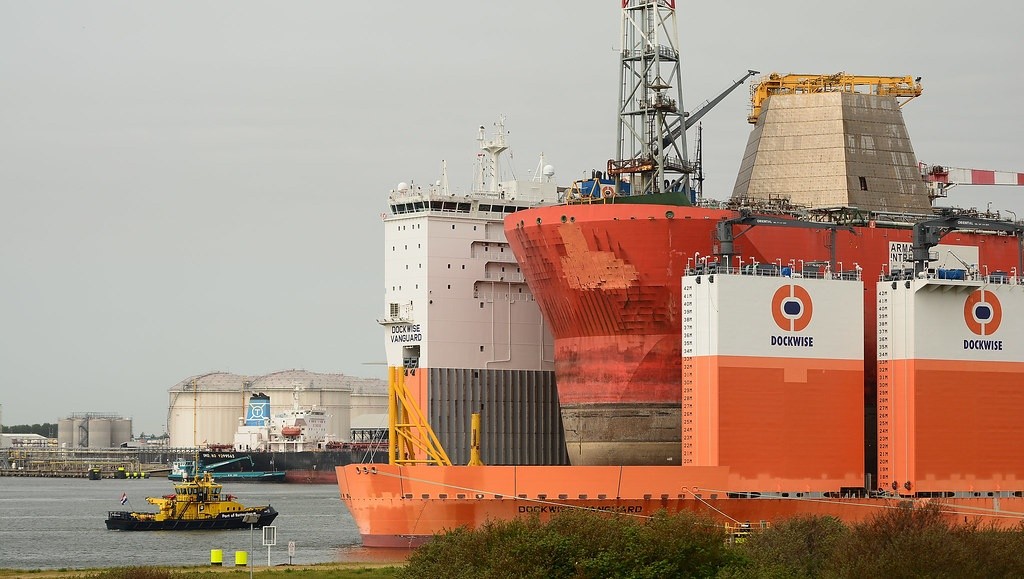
[121,493,128,505]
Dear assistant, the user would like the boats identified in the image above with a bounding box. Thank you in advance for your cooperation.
[197,382,398,484]
[168,458,286,485]
[103,378,278,530]
[281,427,302,436]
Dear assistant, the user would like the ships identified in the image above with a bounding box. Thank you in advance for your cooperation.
[498,89,1024,465]
[331,209,1024,549]
